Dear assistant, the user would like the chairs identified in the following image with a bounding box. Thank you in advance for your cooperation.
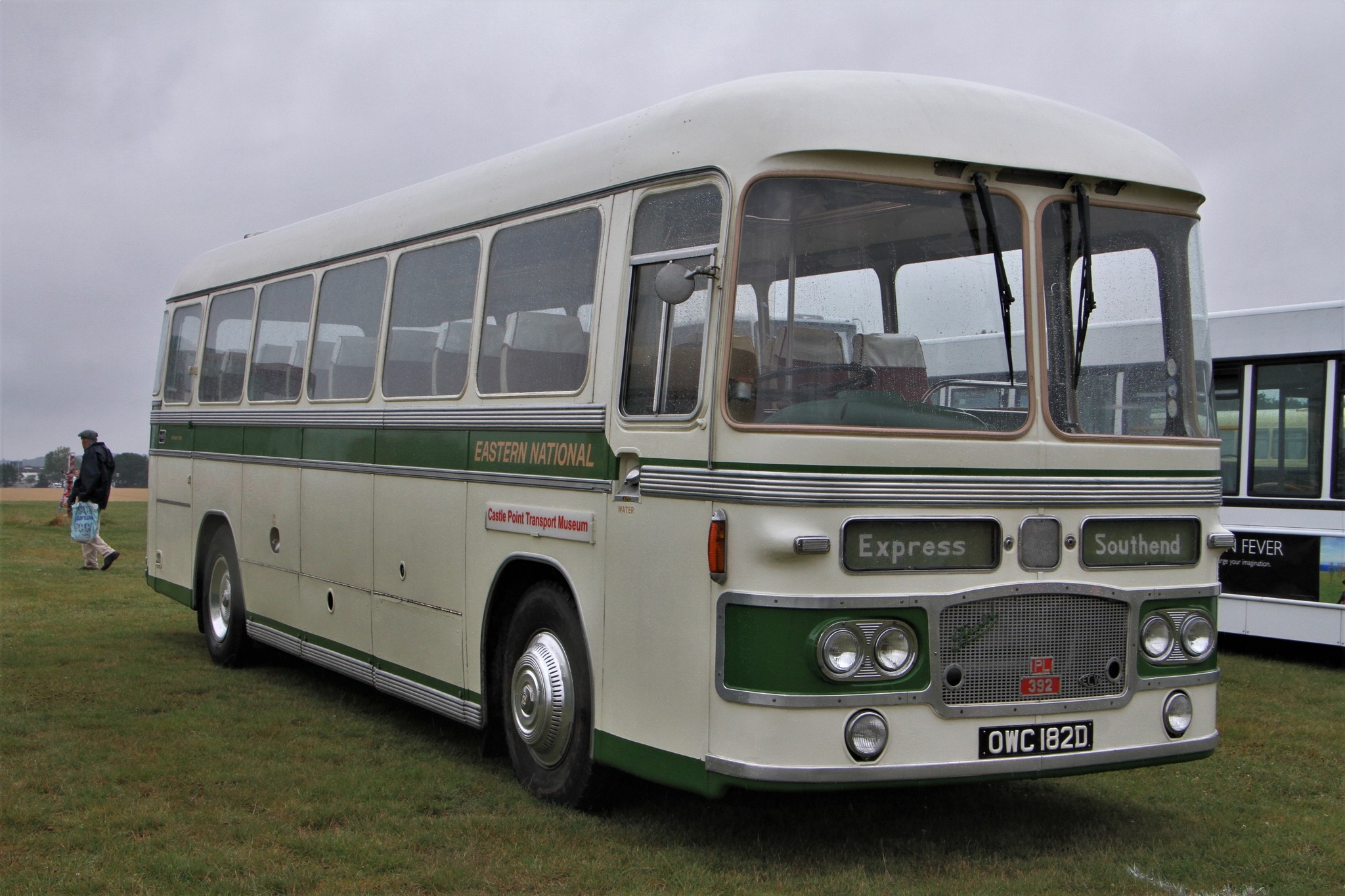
[181,311,928,419]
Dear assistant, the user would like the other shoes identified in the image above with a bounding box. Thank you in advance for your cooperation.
[64,513,72,517]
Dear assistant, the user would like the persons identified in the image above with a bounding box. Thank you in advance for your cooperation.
[67,429,120,571]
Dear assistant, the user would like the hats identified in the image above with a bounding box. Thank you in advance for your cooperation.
[78,429,98,439]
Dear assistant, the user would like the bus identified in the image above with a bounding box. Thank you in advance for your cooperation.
[1151,408,1309,494]
[913,300,1345,652]
[144,70,1234,807]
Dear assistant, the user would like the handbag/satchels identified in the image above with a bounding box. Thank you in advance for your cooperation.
[71,497,98,544]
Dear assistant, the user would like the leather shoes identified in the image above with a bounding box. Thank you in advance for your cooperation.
[77,566,99,571]
[100,551,120,571]
